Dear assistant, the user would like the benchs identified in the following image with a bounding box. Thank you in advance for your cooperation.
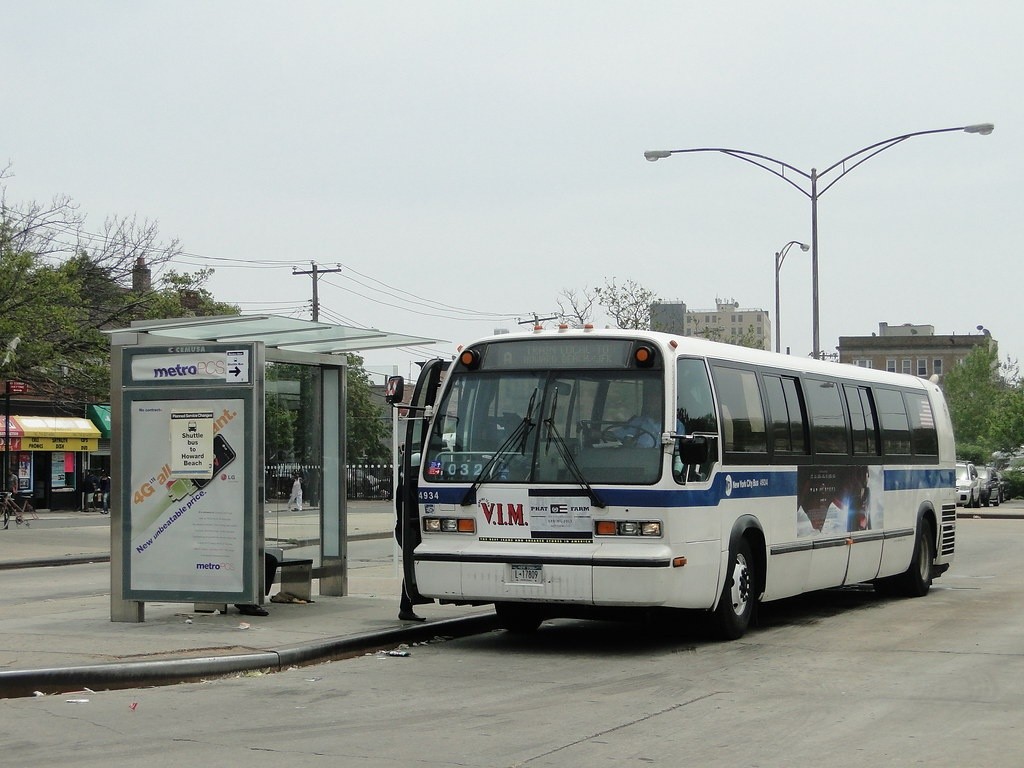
[194,558,313,614]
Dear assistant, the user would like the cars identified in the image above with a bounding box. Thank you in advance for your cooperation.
[956,458,1024,509]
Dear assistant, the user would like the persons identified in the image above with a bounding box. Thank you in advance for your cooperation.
[386,378,398,396]
[8,469,18,515]
[395,453,428,621]
[798,467,871,536]
[83,469,111,514]
[575,385,687,477]
[287,473,302,511]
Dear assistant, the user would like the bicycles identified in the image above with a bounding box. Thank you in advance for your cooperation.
[0,492,39,530]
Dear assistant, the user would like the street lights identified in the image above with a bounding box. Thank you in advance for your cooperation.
[774,241,809,351]
[642,123,995,357]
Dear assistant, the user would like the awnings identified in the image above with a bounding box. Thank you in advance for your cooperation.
[0,415,101,451]
[90,405,111,438]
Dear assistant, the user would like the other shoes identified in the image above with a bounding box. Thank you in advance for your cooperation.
[105,510,108,514]
[100,511,104,514]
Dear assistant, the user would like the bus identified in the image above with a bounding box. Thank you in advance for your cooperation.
[384,324,959,642]
